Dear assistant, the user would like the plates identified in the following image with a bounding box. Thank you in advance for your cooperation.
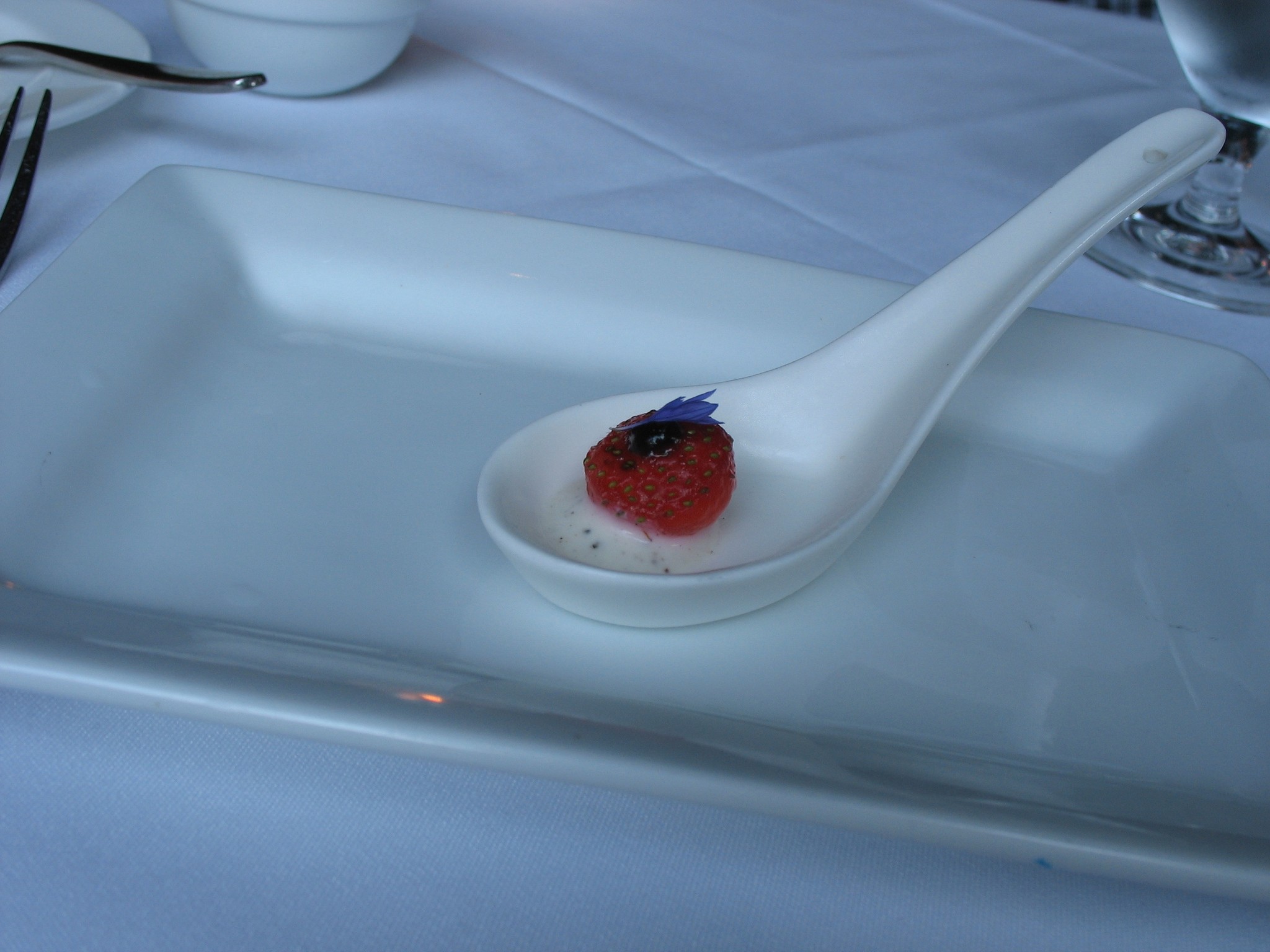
[0,163,1270,903]
[0,0,152,140]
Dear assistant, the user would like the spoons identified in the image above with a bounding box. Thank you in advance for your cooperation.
[477,105,1225,629]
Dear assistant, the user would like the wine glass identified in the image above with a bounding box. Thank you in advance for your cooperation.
[1083,0,1270,316]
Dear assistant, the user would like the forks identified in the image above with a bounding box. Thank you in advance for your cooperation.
[0,87,52,271]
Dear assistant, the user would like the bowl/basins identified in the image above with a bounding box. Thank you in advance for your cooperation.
[174,0,424,97]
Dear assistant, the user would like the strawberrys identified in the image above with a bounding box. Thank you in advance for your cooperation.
[583,410,737,539]
